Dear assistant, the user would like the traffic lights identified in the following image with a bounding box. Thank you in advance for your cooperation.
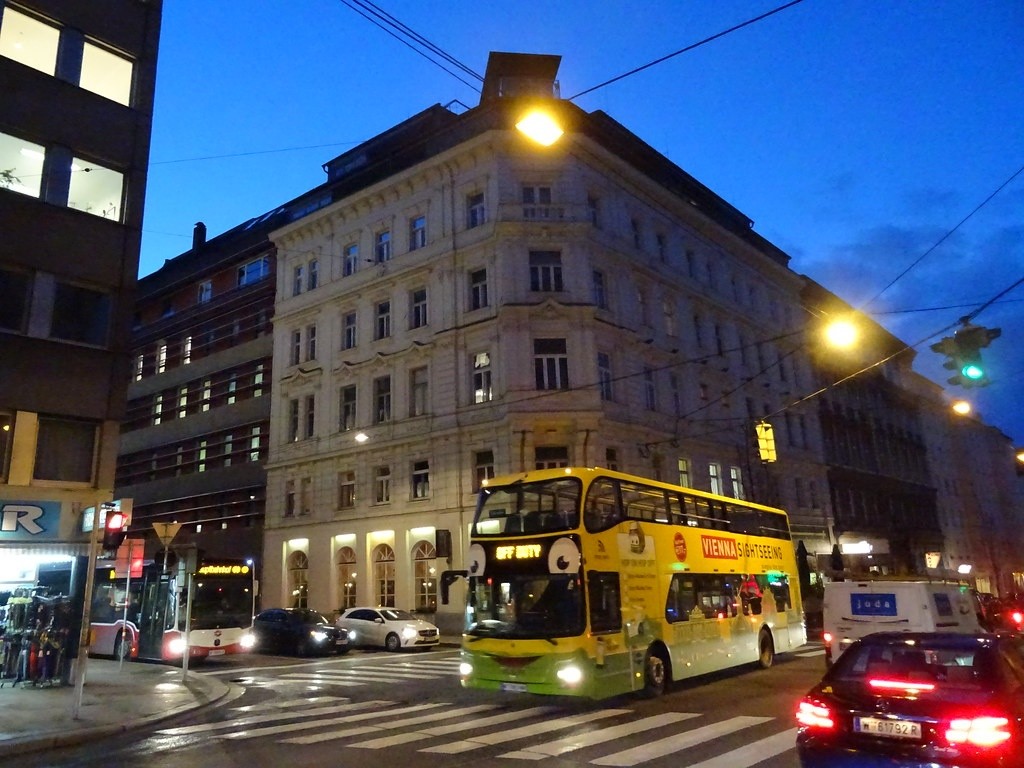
[102,511,128,552]
[756,423,778,465]
[927,326,1001,390]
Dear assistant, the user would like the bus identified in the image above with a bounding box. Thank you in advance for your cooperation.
[439,467,808,699]
[34,556,258,665]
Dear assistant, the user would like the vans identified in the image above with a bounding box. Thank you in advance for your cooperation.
[820,581,990,670]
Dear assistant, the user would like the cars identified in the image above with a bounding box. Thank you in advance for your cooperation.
[789,630,1024,768]
[971,589,1024,632]
[253,608,358,658]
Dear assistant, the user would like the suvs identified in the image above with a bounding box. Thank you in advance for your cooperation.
[335,605,441,652]
